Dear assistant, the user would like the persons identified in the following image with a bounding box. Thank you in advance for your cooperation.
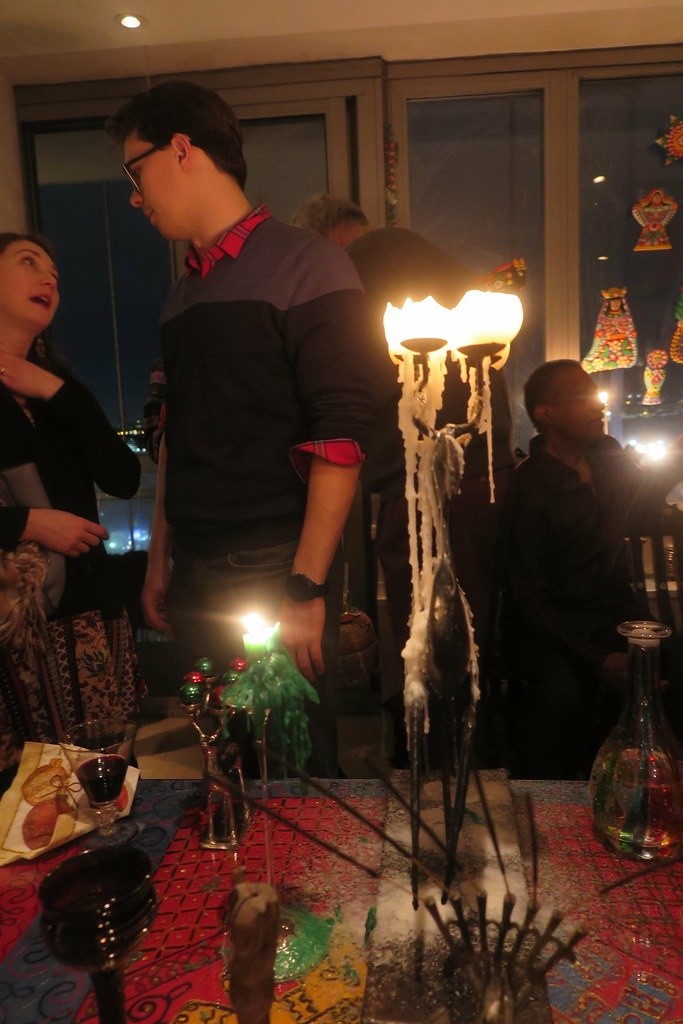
[112,78,381,779]
[293,194,683,783]
[0,234,145,772]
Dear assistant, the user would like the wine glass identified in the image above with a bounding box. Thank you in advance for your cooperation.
[59,718,139,846]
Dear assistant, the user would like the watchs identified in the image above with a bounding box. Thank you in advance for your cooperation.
[278,575,327,603]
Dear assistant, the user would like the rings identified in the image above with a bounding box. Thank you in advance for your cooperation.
[0,368,5,374]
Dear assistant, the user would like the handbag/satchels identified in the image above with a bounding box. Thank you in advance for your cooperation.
[0,607,148,772]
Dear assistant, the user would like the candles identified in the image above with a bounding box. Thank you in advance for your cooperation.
[243,622,281,672]
[383,292,525,373]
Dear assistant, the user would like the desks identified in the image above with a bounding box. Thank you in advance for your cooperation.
[0,777,683,1023]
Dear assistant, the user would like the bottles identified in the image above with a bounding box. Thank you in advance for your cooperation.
[590,621,683,862]
[143,369,168,441]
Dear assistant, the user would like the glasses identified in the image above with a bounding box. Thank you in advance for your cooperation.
[122,140,172,193]
